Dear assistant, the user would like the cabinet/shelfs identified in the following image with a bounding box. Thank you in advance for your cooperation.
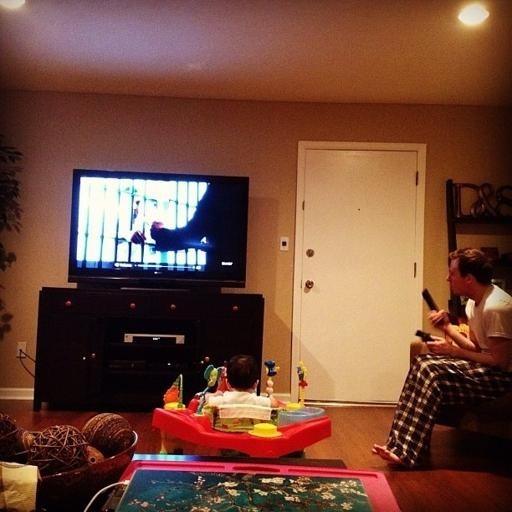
[31,287,266,411]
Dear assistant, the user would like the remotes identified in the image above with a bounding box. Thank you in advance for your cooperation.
[422,289,440,311]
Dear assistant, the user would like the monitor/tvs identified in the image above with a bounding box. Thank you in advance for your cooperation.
[69,168,249,292]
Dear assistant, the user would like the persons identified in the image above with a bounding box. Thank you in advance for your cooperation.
[206,354,280,408]
[149,180,233,270]
[372,245,511,469]
